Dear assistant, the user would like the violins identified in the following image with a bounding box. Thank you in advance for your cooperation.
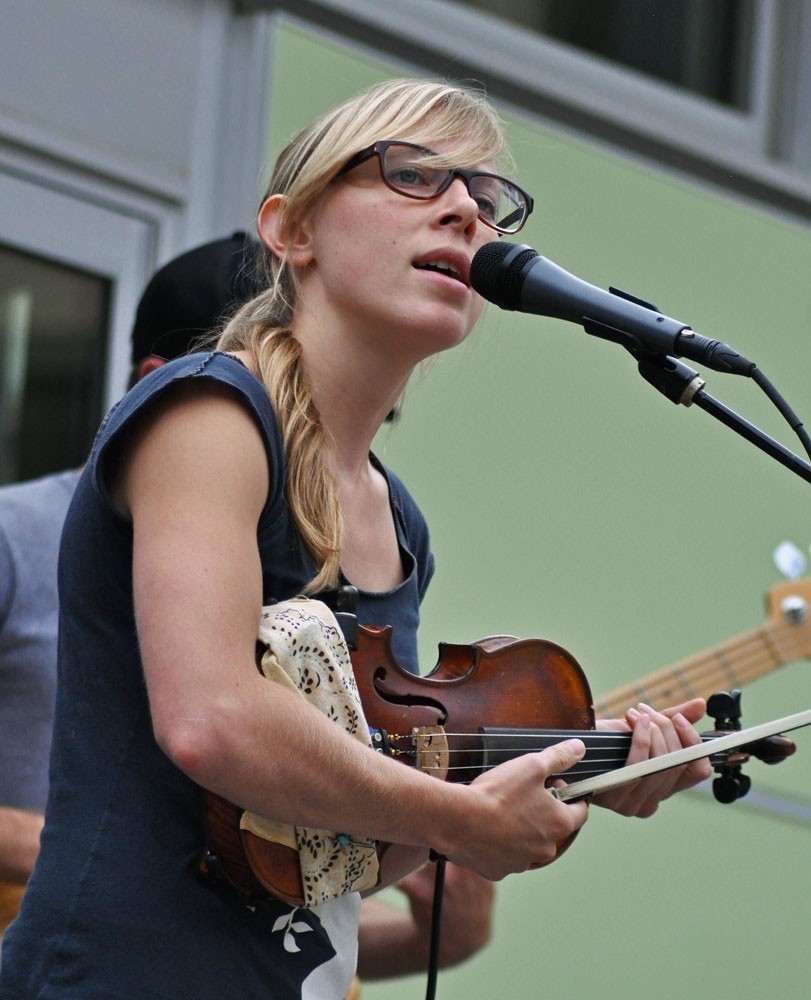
[201,612,797,905]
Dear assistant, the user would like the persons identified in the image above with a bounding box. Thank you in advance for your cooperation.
[0,81,717,1000]
[2,230,495,1000]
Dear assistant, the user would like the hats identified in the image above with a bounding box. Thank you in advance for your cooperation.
[132,230,279,360]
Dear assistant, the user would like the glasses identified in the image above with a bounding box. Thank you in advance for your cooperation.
[329,141,534,238]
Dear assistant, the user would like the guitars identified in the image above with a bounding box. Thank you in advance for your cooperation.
[0,540,811,944]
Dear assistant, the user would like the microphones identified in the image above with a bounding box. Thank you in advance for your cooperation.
[469,239,754,377]
